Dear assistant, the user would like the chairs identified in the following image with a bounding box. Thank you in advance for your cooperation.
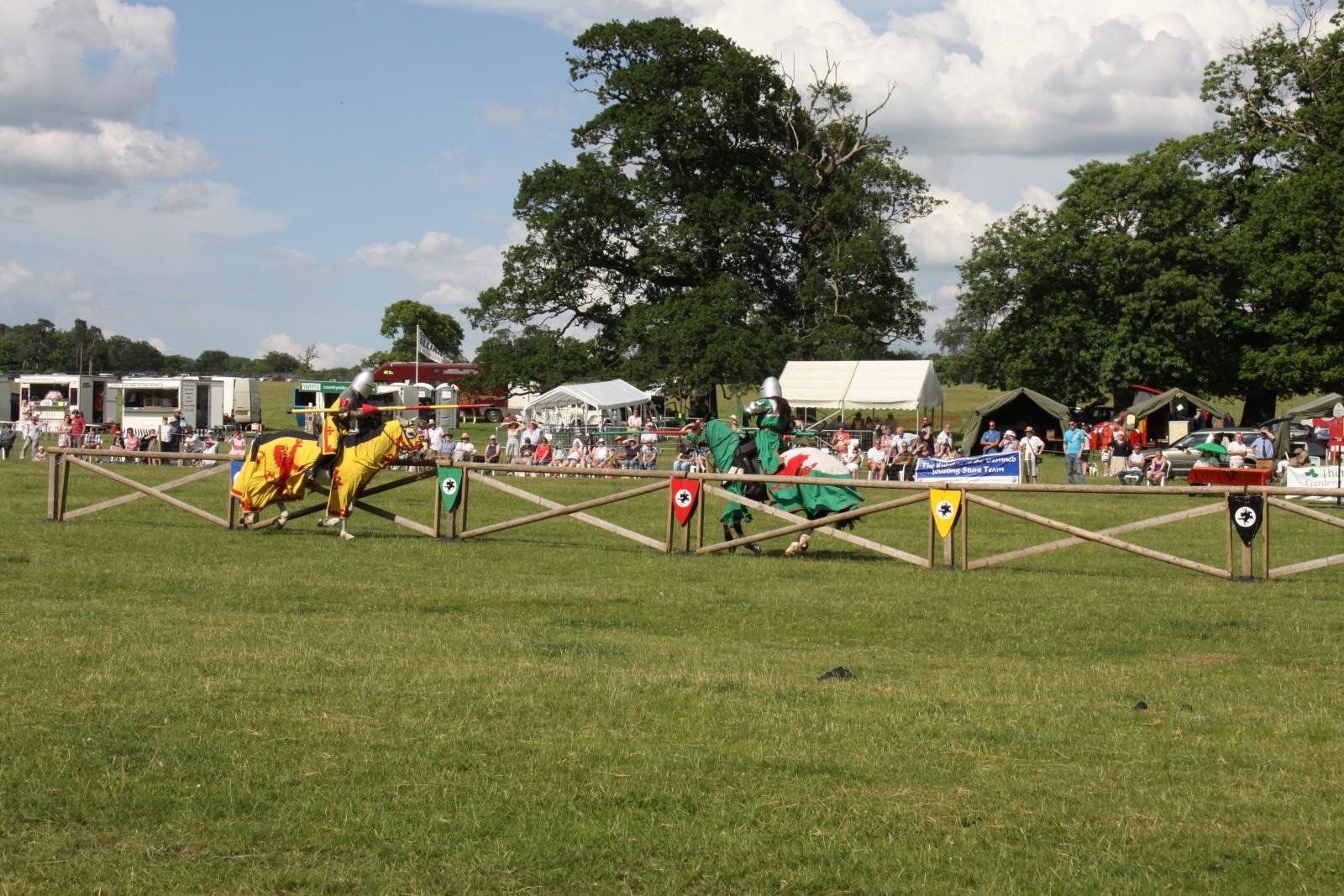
[1124,466,1139,481]
[673,448,711,474]
[509,445,657,478]
[89,439,247,468]
[1147,460,1171,486]
[839,454,908,482]
[1310,456,1320,466]
[245,424,257,439]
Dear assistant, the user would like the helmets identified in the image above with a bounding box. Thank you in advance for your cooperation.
[351,370,375,399]
[761,377,781,397]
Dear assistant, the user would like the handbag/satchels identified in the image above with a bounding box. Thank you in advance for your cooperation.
[1035,455,1043,464]
[1101,449,1111,461]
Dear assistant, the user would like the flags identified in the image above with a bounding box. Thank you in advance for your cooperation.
[418,328,453,365]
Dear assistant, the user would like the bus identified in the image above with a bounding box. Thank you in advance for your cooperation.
[369,360,510,424]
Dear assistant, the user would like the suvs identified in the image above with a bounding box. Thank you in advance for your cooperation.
[1130,414,1296,480]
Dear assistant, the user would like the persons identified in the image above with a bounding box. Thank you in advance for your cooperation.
[392,410,658,479]
[981,421,1045,484]
[793,413,965,481]
[0,423,16,458]
[1281,448,1311,483]
[20,409,263,466]
[1063,419,1167,486]
[1250,427,1276,498]
[720,377,796,488]
[1227,432,1247,468]
[674,415,740,473]
[1192,451,1220,468]
[305,372,381,482]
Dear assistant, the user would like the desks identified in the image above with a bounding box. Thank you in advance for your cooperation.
[24,422,48,441]
[1189,467,1272,486]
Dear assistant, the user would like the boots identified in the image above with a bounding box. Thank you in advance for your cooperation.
[306,454,332,480]
[742,457,761,494]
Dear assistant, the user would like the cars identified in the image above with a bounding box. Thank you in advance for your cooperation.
[256,374,299,382]
[1270,423,1310,440]
[1314,426,1330,441]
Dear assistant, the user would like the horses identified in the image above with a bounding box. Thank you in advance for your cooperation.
[237,414,428,541]
[681,417,862,556]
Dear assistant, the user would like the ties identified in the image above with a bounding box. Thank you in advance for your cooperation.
[1262,439,1266,458]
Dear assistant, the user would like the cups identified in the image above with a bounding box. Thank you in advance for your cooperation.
[309,403,312,407]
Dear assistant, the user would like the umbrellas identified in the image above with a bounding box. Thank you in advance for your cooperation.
[1190,443,1228,455]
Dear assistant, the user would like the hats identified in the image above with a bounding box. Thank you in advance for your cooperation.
[116,431,121,434]
[598,438,604,442]
[490,435,496,439]
[1081,422,1086,425]
[72,409,79,414]
[208,430,213,434]
[174,409,181,413]
[525,437,530,442]
[460,432,470,438]
[1006,430,1016,437]
[958,448,964,453]
[442,431,453,437]
[729,415,739,423]
[429,419,435,425]
[648,422,654,427]
[186,429,195,434]
[838,422,846,427]
[1024,427,1035,433]
[615,436,624,441]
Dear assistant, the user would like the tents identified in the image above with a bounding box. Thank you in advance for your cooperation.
[1274,393,1344,459]
[1123,388,1228,440]
[778,360,945,436]
[961,387,1072,456]
[519,379,651,449]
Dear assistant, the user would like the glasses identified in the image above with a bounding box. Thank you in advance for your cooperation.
[1069,424,1074,426]
